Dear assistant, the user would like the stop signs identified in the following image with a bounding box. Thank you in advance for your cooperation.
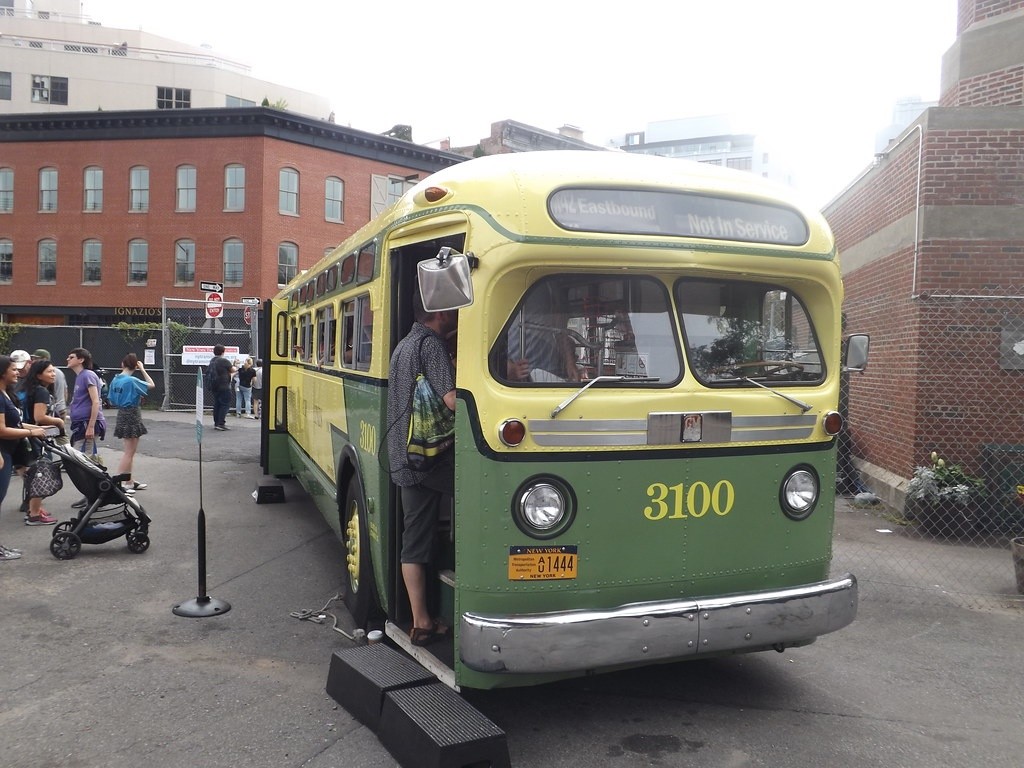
[205,292,224,318]
[244,306,255,325]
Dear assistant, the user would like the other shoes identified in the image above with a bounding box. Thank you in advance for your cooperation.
[121,481,147,489]
[125,489,136,496]
[215,426,230,430]
[0,545,21,559]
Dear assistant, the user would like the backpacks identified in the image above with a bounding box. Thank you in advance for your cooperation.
[205,357,219,392]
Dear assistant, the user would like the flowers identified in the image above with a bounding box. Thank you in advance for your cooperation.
[909,451,987,506]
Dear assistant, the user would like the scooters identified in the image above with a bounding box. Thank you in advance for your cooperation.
[93,369,110,408]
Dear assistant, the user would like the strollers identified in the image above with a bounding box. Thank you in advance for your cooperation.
[32,435,152,560]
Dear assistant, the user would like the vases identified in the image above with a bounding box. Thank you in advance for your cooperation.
[916,499,978,537]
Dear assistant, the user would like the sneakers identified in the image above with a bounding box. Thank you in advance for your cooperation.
[24,510,51,519]
[25,513,58,525]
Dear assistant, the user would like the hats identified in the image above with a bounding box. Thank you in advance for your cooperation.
[10,350,31,369]
[30,349,50,360]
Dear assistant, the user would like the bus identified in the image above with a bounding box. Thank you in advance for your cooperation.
[268,149,870,693]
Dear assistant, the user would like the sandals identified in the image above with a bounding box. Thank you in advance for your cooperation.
[410,619,454,646]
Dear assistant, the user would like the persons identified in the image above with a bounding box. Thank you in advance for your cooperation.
[233,358,257,419]
[24,360,65,526]
[10,350,33,476]
[67,348,106,508]
[387,289,457,646]
[252,359,263,419]
[107,353,155,493]
[30,349,69,472]
[506,292,581,382]
[209,344,237,430]
[0,354,46,513]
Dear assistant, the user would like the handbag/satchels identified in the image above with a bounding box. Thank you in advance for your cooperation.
[405,334,454,472]
[81,437,103,466]
[23,455,62,497]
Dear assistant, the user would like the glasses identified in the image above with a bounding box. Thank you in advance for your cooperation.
[68,356,78,359]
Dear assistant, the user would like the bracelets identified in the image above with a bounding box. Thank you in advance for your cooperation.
[29,429,32,434]
